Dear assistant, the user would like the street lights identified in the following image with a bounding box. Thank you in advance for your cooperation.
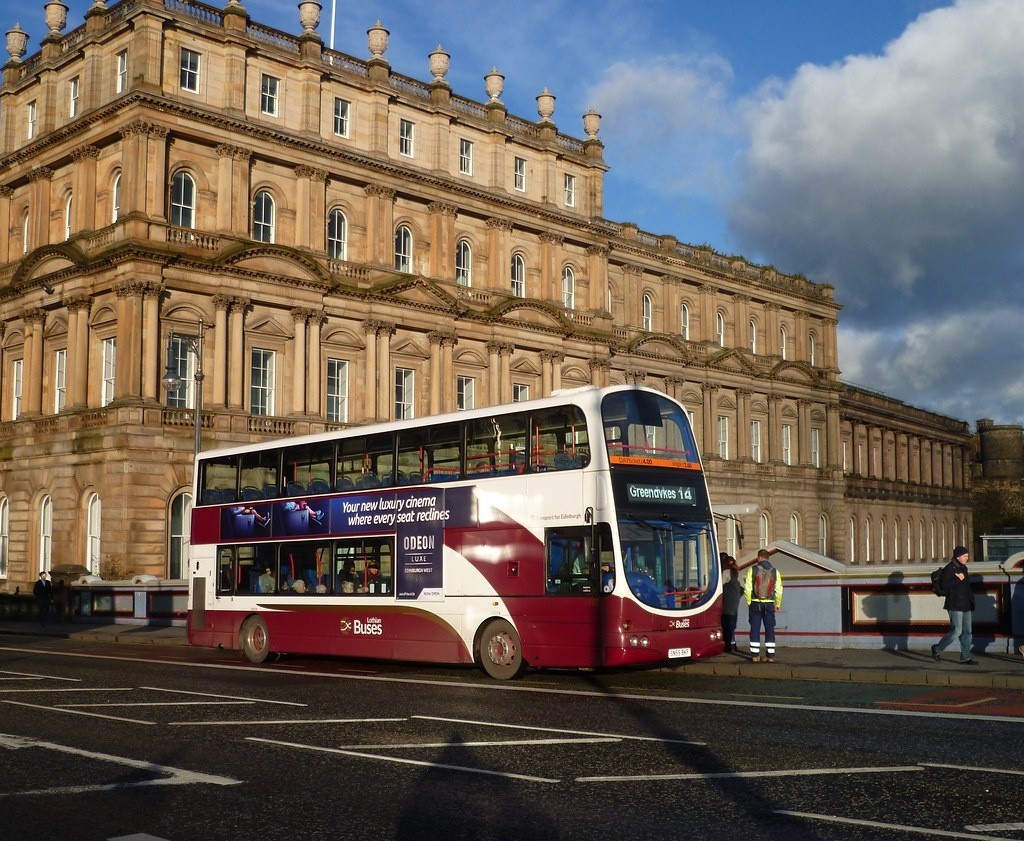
[160,318,207,453]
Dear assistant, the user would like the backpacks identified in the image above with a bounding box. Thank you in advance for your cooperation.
[753,564,775,599]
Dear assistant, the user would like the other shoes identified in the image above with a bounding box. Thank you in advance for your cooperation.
[931,645,940,662]
[761,656,774,662]
[960,659,979,665]
[753,656,760,662]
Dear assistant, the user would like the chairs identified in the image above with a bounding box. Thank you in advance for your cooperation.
[202,451,590,505]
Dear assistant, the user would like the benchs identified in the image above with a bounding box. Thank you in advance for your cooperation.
[222,564,365,594]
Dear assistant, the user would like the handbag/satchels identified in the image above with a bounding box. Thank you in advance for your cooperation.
[930,564,956,597]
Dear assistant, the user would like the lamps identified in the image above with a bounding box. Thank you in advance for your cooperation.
[40,283,51,293]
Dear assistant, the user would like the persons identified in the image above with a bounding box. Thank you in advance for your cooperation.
[681,586,701,608]
[33,571,142,628]
[571,550,609,596]
[259,559,391,593]
[931,545,980,666]
[744,549,784,663]
[720,552,745,653]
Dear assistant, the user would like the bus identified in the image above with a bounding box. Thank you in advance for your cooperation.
[185,384,748,680]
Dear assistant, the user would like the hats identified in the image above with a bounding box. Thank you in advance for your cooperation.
[368,560,379,569]
[953,545,968,558]
